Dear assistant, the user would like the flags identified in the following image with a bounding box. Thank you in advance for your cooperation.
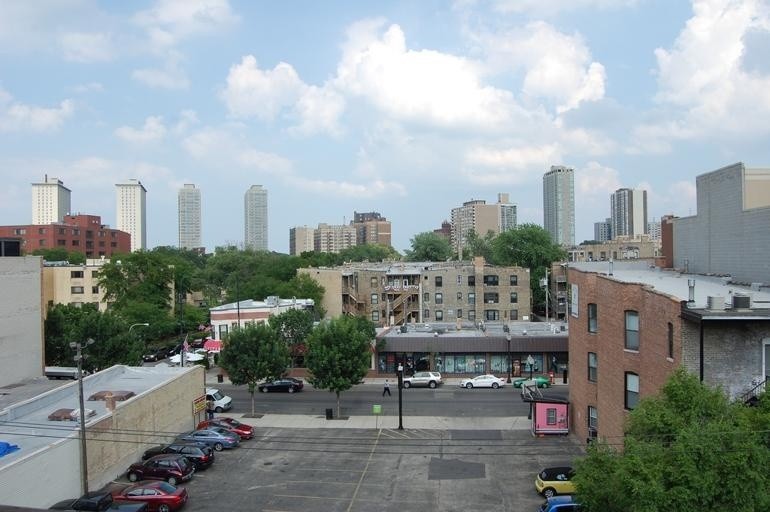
[184,335,190,352]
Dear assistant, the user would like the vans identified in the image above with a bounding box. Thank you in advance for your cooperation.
[205,387,233,412]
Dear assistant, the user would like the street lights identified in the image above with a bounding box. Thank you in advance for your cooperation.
[224,273,241,332]
[128,323,149,334]
[396,362,404,430]
[69,338,96,496]
[507,334,513,384]
[180,274,198,340]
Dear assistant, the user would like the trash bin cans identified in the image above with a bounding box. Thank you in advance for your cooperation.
[326,408,333,420]
[218,374,223,383]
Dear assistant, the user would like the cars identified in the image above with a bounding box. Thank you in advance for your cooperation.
[458,374,505,390]
[534,467,585,511]
[257,377,304,394]
[513,375,552,389]
[142,338,208,363]
[46,417,255,511]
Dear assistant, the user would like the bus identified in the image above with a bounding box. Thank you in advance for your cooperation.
[45,366,90,380]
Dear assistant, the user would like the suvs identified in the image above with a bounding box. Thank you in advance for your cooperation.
[403,371,442,389]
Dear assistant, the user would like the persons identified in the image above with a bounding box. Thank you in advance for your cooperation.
[382,378,391,397]
[206,398,215,419]
